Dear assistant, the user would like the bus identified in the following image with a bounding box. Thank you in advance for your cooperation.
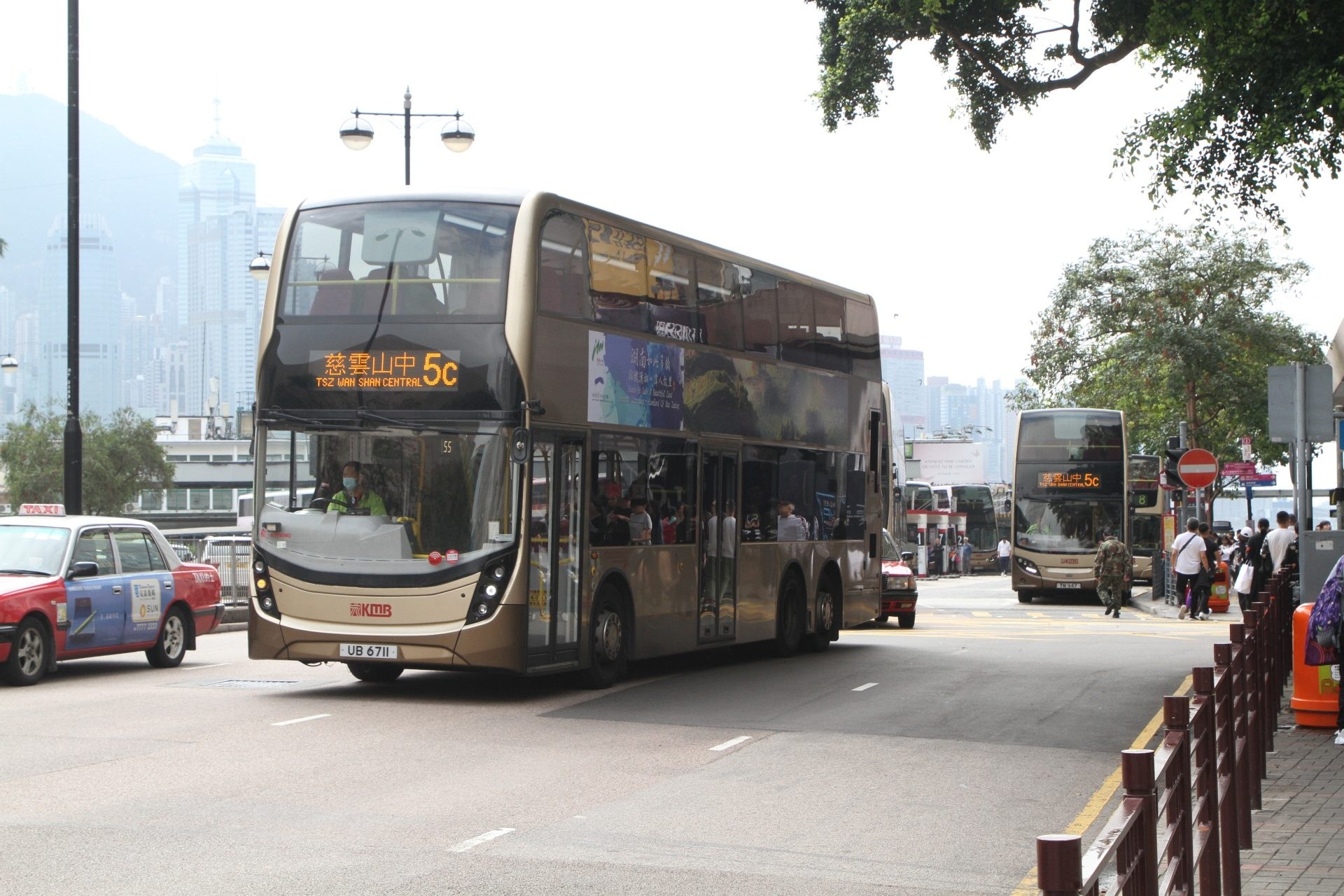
[1004,408,1164,603]
[246,190,896,693]
[904,481,1000,575]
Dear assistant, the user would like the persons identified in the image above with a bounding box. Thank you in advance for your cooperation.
[996,536,1011,576]
[1093,526,1133,618]
[1026,514,1052,536]
[560,471,846,613]
[962,536,972,574]
[1260,510,1298,574]
[947,542,959,573]
[327,460,388,517]
[1170,516,1216,621]
[927,537,945,575]
[1170,510,1332,621]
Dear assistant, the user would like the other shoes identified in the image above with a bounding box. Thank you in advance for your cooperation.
[1113,613,1119,618]
[1199,611,1206,621]
[1334,728,1344,744]
[1178,605,1187,620]
[1105,602,1115,614]
[1206,608,1212,619]
[1001,572,1007,576]
[1189,614,1199,620]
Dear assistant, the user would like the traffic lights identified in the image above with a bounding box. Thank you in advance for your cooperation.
[1163,448,1190,489]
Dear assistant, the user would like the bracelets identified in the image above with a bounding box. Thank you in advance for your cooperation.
[1208,567,1211,571]
[1171,567,1176,569]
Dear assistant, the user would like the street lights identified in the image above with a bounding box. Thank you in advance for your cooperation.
[248,245,337,508]
[337,85,477,186]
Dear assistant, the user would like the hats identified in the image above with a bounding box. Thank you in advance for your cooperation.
[1240,526,1253,538]
[1104,527,1113,535]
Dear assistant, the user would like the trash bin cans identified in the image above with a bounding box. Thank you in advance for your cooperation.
[1290,603,1340,727]
[1208,562,1230,612]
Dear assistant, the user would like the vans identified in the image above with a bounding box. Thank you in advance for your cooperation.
[171,536,253,595]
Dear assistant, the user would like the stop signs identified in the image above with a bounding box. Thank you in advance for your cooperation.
[1177,448,1219,489]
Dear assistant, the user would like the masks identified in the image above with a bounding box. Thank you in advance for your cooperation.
[343,478,357,488]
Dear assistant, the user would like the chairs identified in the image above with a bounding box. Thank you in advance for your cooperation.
[540,267,588,318]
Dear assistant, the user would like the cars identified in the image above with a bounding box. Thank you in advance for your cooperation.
[881,528,918,629]
[0,503,225,686]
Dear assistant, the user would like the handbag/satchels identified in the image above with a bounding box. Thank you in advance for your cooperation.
[1304,555,1344,666]
[1235,559,1254,594]
[951,554,956,562]
[955,555,960,563]
[1186,589,1192,608]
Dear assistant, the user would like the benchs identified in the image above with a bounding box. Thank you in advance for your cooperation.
[309,266,412,316]
